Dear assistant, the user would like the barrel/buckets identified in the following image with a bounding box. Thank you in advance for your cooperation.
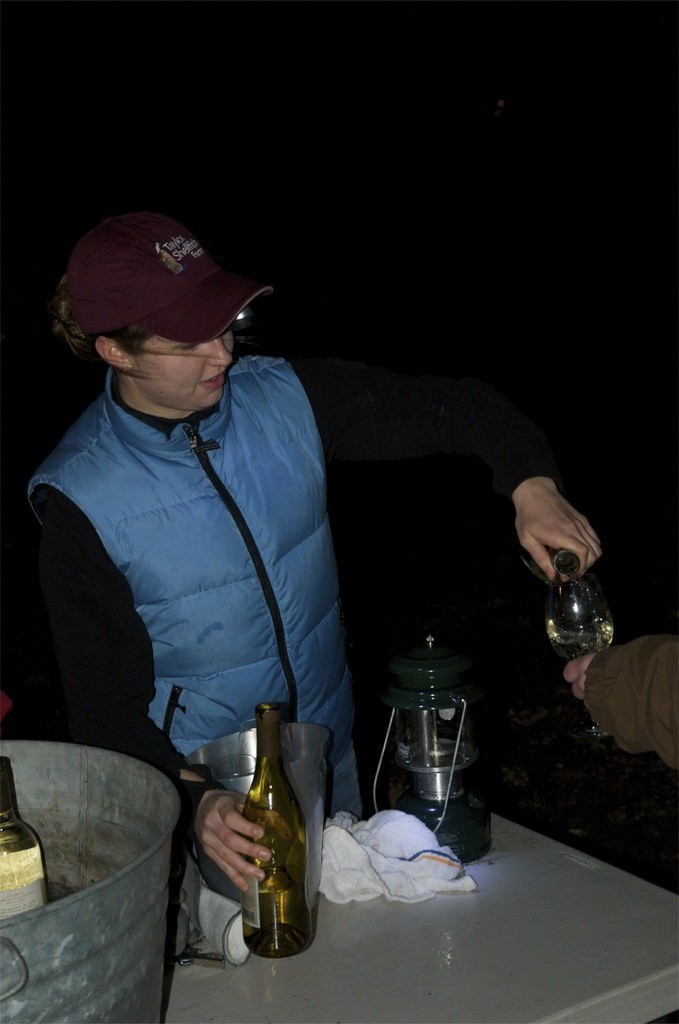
[1,739,182,1024]
[186,719,333,912]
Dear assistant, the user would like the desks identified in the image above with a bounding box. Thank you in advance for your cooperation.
[159,800,678,1022]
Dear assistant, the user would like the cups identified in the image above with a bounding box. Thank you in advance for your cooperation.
[213,753,258,779]
[238,716,297,767]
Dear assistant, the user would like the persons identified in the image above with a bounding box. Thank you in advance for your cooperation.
[559,633,678,776]
[30,210,603,901]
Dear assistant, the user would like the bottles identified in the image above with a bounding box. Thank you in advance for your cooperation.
[239,701,312,958]
[518,544,578,584]
[0,755,48,924]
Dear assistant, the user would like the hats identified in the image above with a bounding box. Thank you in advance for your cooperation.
[66,212,274,344]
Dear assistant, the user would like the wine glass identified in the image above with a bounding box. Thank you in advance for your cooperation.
[544,572,620,736]
